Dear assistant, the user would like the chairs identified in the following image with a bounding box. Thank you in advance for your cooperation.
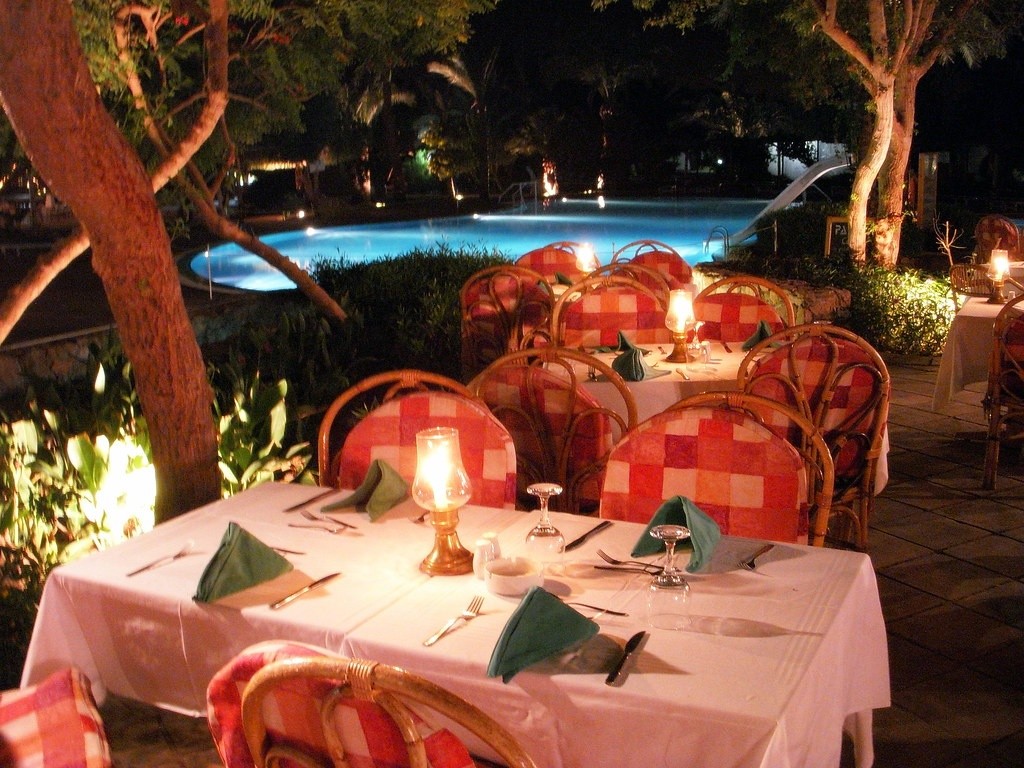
[738,323,891,551]
[466,349,639,515]
[951,262,1005,311]
[516,241,601,279]
[318,370,517,512]
[553,277,670,344]
[598,394,835,551]
[985,294,1024,493]
[460,266,554,362]
[692,276,796,342]
[973,215,1019,261]
[205,638,534,767]
[612,238,692,288]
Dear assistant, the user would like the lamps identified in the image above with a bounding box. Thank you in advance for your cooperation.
[989,248,1008,304]
[577,242,596,289]
[665,287,698,364]
[412,426,474,574]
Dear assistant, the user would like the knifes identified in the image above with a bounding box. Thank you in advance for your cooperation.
[605,631,646,686]
[270,573,341,609]
[720,342,732,353]
[565,521,612,553]
[676,367,689,379]
[282,489,341,512]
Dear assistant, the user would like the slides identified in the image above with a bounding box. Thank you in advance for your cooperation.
[702,153,854,248]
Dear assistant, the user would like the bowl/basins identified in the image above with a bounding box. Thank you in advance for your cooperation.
[484,557,544,595]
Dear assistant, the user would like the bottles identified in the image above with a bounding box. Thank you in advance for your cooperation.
[473,540,494,579]
[483,532,500,558]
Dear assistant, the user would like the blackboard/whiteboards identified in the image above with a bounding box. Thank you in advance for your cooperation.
[825,215,876,258]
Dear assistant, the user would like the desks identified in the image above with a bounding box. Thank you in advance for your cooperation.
[979,262,1024,292]
[545,343,890,495]
[21,479,892,768]
[933,298,1024,414]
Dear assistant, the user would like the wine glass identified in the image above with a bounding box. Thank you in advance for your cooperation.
[687,321,707,369]
[525,483,565,576]
[647,524,692,630]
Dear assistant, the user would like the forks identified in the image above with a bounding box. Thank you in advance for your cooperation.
[587,365,597,382]
[409,509,430,523]
[288,523,349,534]
[128,541,194,578]
[301,510,358,530]
[424,596,484,646]
[737,544,774,570]
[596,548,664,569]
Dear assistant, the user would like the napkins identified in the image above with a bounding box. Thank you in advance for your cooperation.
[740,320,780,349]
[613,350,673,380]
[193,520,291,601]
[598,330,651,356]
[323,461,410,516]
[486,583,600,681]
[631,497,721,574]
